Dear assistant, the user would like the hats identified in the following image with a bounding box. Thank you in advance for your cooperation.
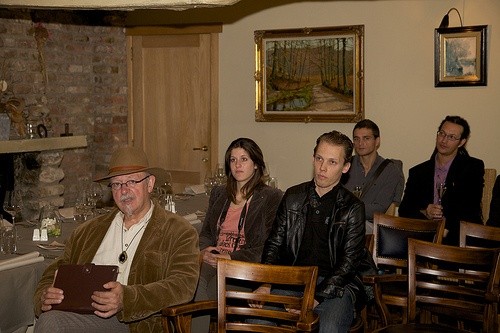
[92,147,151,187]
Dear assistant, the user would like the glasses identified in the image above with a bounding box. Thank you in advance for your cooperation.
[108,175,149,191]
[437,131,461,141]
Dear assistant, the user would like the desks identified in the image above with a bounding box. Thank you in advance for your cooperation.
[0,188,283,333]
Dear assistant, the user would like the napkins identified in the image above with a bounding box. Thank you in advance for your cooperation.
[184,184,207,195]
[54,207,93,221]
[36,241,65,250]
[183,212,202,225]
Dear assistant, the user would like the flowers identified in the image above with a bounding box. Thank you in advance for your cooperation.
[39,217,61,238]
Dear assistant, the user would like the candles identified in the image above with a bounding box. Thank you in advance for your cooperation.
[0,250,40,266]
[0,255,44,272]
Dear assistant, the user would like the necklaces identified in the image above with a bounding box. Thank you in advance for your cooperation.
[118,215,149,264]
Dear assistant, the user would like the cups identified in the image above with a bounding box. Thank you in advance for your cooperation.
[26,123,35,139]
[351,186,362,199]
[263,163,278,189]
[204,166,225,198]
[0,226,17,255]
[160,194,175,213]
[73,203,87,223]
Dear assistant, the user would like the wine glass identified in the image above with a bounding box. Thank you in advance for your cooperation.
[3,190,25,226]
[436,182,447,205]
[86,183,104,218]
[159,171,172,203]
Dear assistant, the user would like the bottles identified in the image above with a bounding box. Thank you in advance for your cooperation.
[38,204,62,237]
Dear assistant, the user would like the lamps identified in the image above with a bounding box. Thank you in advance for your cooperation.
[438,7,462,34]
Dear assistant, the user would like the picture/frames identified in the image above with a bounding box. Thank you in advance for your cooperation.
[253,24,365,124]
[433,24,488,88]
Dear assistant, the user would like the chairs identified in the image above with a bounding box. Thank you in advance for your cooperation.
[368,210,447,324]
[358,237,500,333]
[481,169,497,226]
[161,257,320,333]
[458,220,500,333]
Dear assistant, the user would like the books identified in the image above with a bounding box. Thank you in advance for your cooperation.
[49,263,119,314]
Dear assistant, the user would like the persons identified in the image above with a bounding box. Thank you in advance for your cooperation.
[342,118,401,236]
[32,145,201,333]
[398,115,485,290]
[190,137,284,333]
[244,129,366,333]
